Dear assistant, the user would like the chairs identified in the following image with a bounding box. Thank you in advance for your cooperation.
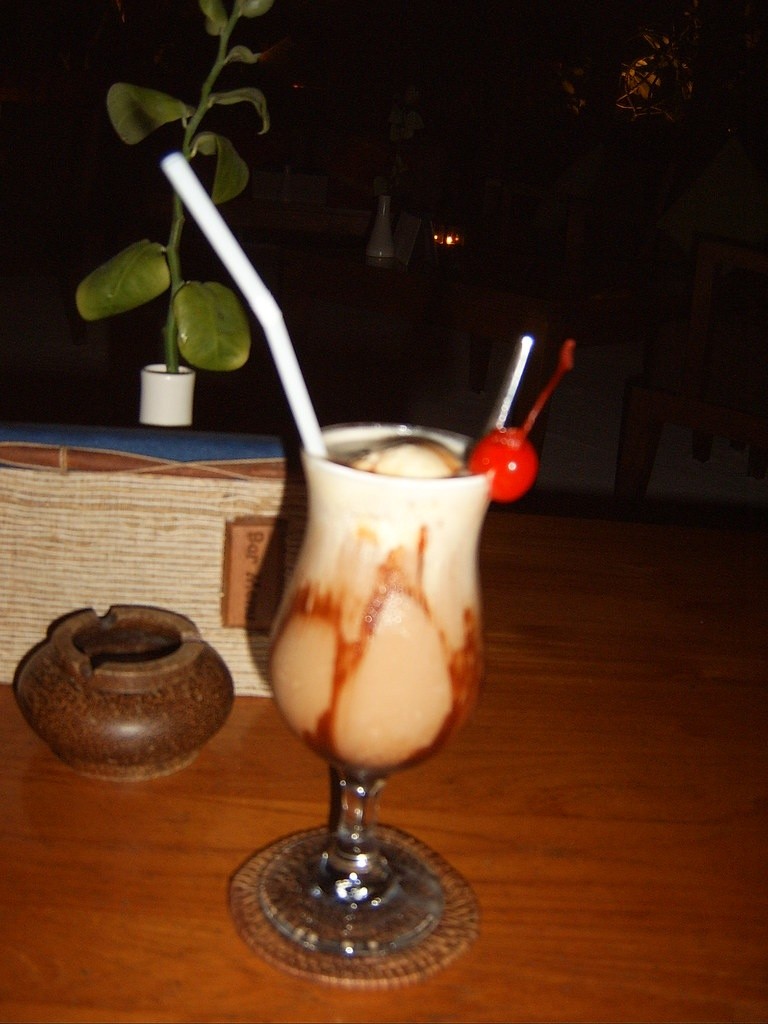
[53,193,767,514]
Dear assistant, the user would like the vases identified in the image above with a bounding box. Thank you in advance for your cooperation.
[136,363,195,427]
[365,195,396,269]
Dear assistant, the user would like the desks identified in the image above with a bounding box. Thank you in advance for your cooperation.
[1,511,768,1024]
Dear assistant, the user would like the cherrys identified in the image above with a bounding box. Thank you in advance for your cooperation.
[468,338,575,501]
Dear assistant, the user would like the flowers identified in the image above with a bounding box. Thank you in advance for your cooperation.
[374,88,422,196]
[75,0,274,372]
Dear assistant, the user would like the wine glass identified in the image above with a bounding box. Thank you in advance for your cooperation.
[226,426,495,988]
